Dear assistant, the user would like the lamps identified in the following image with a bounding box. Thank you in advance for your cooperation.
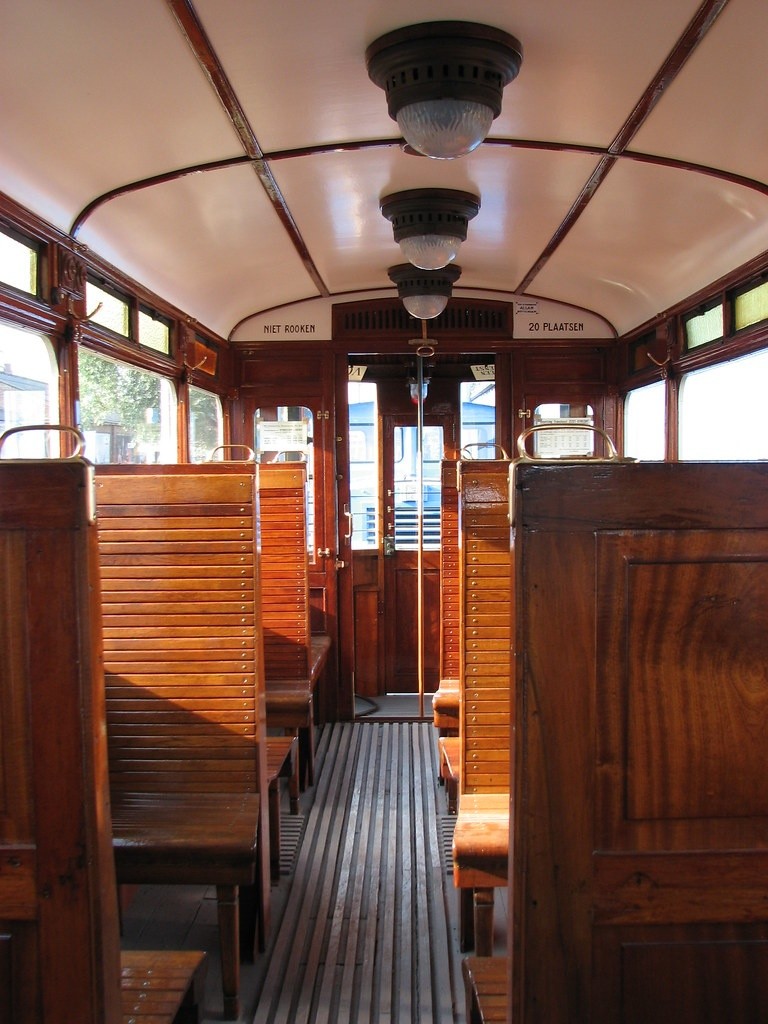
[380,187,482,270]
[366,19,524,160]
[387,262,462,319]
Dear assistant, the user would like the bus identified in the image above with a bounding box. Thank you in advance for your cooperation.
[308,402,499,552]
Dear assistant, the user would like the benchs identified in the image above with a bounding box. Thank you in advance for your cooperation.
[0,423,768,1024]
